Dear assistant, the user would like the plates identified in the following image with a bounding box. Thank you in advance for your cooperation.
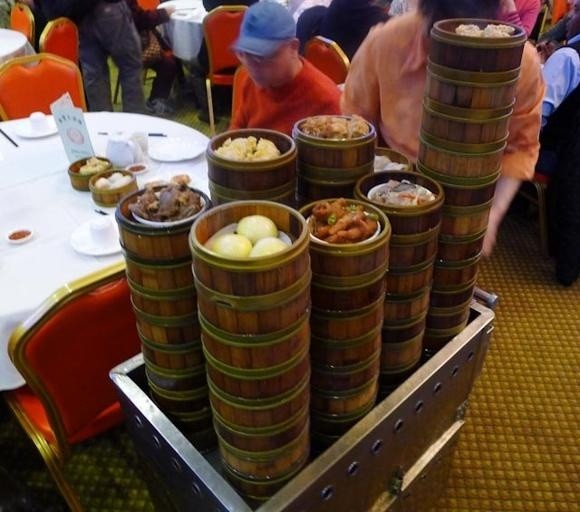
[8,231,32,247]
[366,181,434,210]
[203,220,294,259]
[124,160,150,175]
[73,226,124,261]
[15,126,58,140]
[143,126,208,163]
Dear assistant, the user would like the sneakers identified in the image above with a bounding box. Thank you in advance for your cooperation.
[145,100,174,115]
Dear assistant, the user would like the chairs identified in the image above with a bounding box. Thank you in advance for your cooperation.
[297,31,350,83]
[39,17,80,69]
[203,3,251,138]
[57,460,60,462]
[113,1,179,109]
[9,3,35,45]
[524,171,552,279]
[0,52,90,123]
[3,259,141,510]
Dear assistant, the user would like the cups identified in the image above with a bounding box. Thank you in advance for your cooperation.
[91,217,115,245]
[131,132,150,163]
[26,112,48,135]
[106,130,132,168]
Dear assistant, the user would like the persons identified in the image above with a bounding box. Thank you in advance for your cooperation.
[33,0,257,122]
[296,1,580,260]
[227,0,341,135]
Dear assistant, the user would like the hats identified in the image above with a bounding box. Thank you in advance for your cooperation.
[231,1,296,56]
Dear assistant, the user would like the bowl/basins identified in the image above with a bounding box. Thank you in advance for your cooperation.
[305,210,382,248]
[133,189,207,228]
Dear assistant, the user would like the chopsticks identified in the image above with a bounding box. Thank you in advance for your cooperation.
[0,129,18,151]
[91,129,168,140]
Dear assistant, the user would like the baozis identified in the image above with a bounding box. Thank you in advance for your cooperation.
[92,171,130,190]
[213,215,284,259]
[80,157,109,174]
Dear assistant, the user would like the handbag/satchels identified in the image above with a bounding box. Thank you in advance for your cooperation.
[142,31,160,62]
